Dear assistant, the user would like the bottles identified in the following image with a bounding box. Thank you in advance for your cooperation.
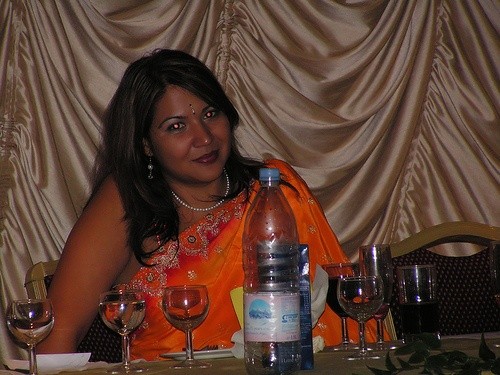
[242,167,302,375]
[360,244,400,351]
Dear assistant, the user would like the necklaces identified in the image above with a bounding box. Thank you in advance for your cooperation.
[168,166,231,211]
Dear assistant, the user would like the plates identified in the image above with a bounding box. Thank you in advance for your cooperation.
[159,348,234,360]
[36,352,91,367]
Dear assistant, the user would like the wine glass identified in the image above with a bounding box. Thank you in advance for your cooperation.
[6,299,54,375]
[162,284,212,369]
[337,276,383,360]
[322,263,361,350]
[98,291,146,373]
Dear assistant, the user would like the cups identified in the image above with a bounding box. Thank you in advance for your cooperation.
[396,264,441,343]
[488,241,500,307]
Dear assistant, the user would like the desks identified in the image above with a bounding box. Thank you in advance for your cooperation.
[36,331,500,375]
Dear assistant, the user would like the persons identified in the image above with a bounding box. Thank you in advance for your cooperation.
[34,47,391,361]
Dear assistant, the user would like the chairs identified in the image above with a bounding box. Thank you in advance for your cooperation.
[374,221,500,340]
[25,260,122,363]
